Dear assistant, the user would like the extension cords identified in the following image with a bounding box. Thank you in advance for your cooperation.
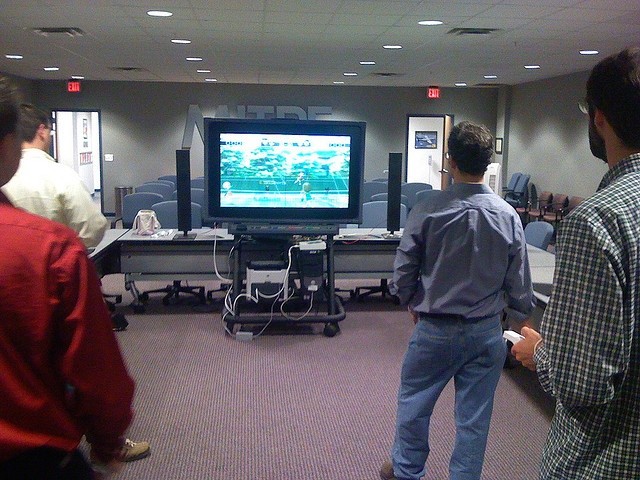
[298,240,327,250]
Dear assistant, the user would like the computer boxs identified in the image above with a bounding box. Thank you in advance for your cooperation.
[298,245,323,304]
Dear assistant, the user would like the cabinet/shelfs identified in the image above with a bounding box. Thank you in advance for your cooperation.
[222,225,347,341]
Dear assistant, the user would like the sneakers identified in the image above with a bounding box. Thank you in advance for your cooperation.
[379,462,421,480]
[91,436,150,461]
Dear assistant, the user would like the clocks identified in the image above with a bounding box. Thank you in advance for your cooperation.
[494,138,502,153]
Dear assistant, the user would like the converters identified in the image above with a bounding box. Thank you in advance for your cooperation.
[235,331,254,343]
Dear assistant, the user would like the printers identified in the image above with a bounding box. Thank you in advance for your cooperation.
[245,249,288,304]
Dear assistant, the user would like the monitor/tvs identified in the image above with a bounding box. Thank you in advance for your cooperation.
[206,118,367,236]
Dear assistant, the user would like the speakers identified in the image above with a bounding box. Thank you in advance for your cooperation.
[381,151,402,240]
[171,150,198,240]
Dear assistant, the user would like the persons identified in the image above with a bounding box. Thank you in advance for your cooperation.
[0,73,135,480]
[378,120,538,479]
[508,46,639,479]
[3,101,150,463]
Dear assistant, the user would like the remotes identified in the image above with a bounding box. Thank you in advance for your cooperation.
[501,330,527,347]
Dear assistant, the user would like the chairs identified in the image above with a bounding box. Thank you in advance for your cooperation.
[158,175,178,182]
[172,189,204,201]
[121,194,162,228]
[524,220,555,251]
[143,180,174,192]
[503,173,587,228]
[191,178,205,189]
[402,183,433,194]
[413,189,442,210]
[363,182,388,203]
[352,200,408,303]
[372,178,388,182]
[137,185,169,200]
[141,202,204,306]
[370,193,406,204]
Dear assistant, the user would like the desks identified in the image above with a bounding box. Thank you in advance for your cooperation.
[121,229,402,314]
[505,244,555,336]
[88,227,129,330]
